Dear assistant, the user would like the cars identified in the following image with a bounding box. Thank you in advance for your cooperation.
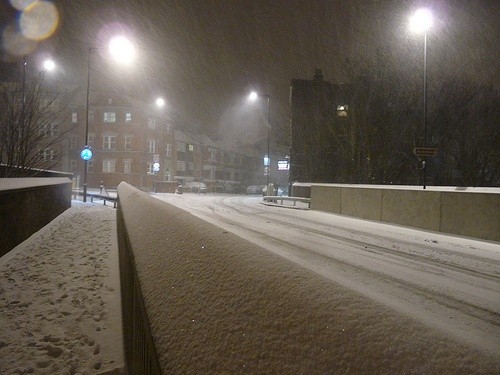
[153,177,290,199]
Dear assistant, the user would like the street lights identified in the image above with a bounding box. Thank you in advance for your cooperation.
[246,92,271,202]
[157,96,177,187]
[79,35,144,207]
[19,55,55,169]
[408,8,435,189]
